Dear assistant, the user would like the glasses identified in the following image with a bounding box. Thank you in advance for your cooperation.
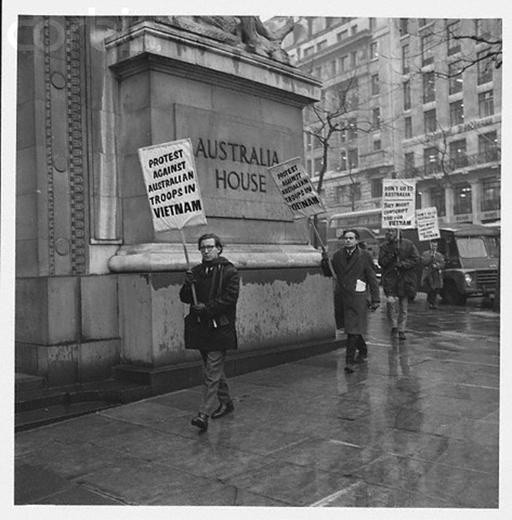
[199,246,215,251]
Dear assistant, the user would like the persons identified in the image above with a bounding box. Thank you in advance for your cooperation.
[321,228,382,373]
[377,228,419,341]
[179,232,241,430]
[419,240,446,310]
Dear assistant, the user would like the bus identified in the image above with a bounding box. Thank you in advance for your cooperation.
[325,206,500,305]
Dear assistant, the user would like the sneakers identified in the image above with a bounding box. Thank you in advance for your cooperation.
[392,328,406,339]
[192,417,208,429]
[212,402,234,418]
[345,355,367,371]
